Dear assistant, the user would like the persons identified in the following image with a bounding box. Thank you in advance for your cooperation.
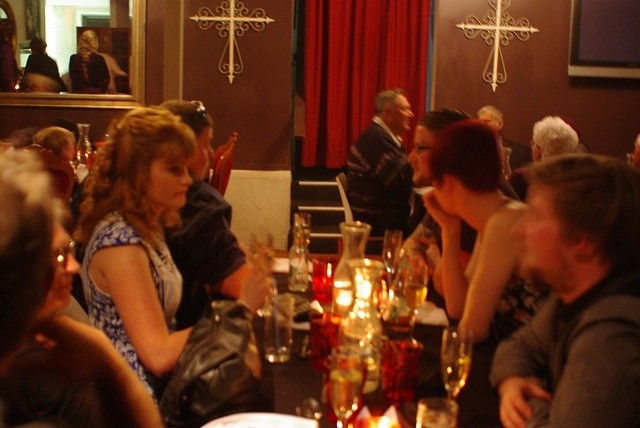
[508,115,580,201]
[477,105,531,180]
[489,152,637,427]
[160,100,246,329]
[347,88,425,239]
[401,107,521,287]
[80,107,198,407]
[1,146,54,359]
[0,196,126,427]
[19,39,67,92]
[422,118,551,344]
[69,30,110,94]
[33,126,81,217]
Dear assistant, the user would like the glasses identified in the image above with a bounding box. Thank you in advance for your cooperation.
[190,101,208,125]
[53,239,75,266]
[416,143,432,155]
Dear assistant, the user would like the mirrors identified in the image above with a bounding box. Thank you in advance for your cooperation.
[0,0,146,111]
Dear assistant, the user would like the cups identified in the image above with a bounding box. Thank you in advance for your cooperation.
[263,296,296,362]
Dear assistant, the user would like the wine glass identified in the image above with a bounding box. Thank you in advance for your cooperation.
[440,330,474,402]
[289,209,312,267]
[402,265,429,348]
[327,348,363,427]
[382,229,402,287]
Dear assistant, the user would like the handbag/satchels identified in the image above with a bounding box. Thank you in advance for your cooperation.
[156,300,273,428]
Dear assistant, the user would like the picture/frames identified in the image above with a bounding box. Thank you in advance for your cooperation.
[566,1,640,80]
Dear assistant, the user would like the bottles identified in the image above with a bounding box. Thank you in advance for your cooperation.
[76,122,92,161]
[332,218,373,325]
[340,259,384,395]
[289,223,308,292]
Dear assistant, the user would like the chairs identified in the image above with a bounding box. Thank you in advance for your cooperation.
[336,172,403,256]
